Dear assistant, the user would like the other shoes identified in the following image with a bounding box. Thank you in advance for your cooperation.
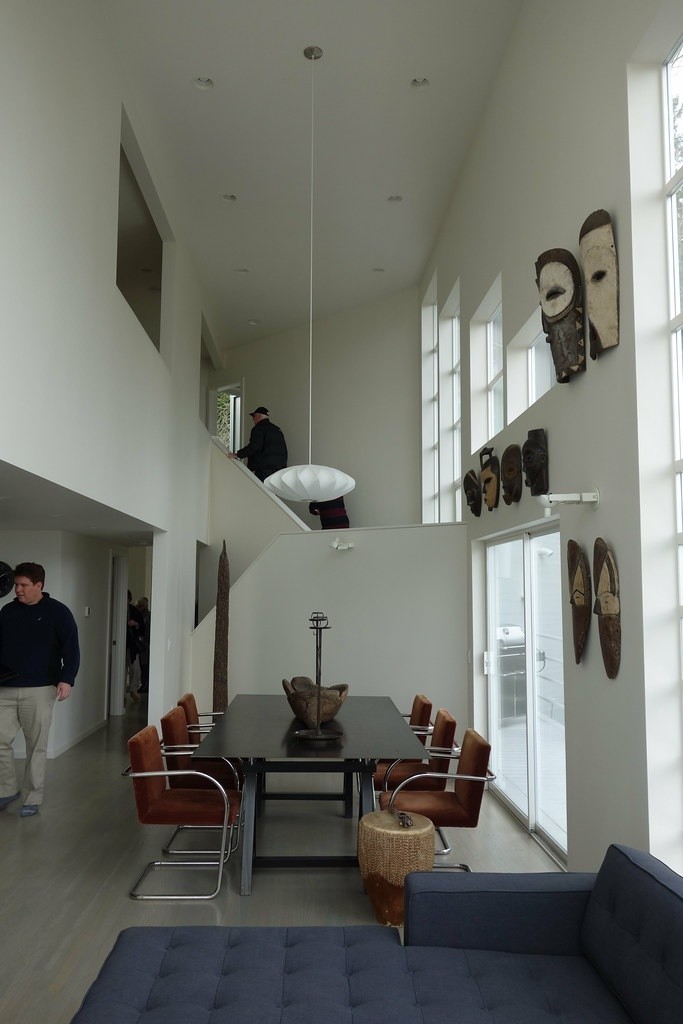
[137,685,148,693]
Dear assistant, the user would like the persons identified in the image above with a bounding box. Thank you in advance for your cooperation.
[135,597,151,694]
[0,562,80,817]
[126,589,142,703]
[309,496,349,530]
[227,407,288,496]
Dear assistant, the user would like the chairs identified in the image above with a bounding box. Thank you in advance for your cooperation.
[119,693,495,900]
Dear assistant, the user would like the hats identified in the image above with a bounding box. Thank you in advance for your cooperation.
[249,407,269,416]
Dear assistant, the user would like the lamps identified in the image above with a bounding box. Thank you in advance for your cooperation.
[264,46,356,504]
[329,538,355,551]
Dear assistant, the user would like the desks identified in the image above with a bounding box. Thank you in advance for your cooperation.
[188,692,434,896]
[360,810,433,926]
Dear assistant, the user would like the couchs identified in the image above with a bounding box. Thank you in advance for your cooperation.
[72,844,683,1024]
